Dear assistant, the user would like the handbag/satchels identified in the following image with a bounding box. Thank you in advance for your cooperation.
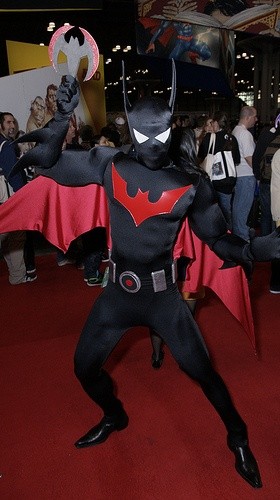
[212,150,238,186]
[198,153,213,182]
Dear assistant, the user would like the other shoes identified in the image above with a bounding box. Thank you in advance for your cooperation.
[269,279,280,294]
[57,257,76,265]
[26,265,36,273]
[25,274,38,282]
[83,274,104,287]
[103,255,109,262]
[152,349,165,369]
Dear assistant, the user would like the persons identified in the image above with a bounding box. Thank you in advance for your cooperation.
[268,149,279,294]
[7,59,280,488]
[149,126,201,370]
[170,110,274,142]
[229,106,259,242]
[258,111,280,236]
[1,110,38,285]
[55,117,137,287]
[195,111,241,234]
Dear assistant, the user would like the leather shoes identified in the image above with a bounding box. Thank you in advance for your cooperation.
[74,412,128,448]
[227,434,263,488]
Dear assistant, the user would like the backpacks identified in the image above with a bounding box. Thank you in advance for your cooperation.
[0,167,15,203]
